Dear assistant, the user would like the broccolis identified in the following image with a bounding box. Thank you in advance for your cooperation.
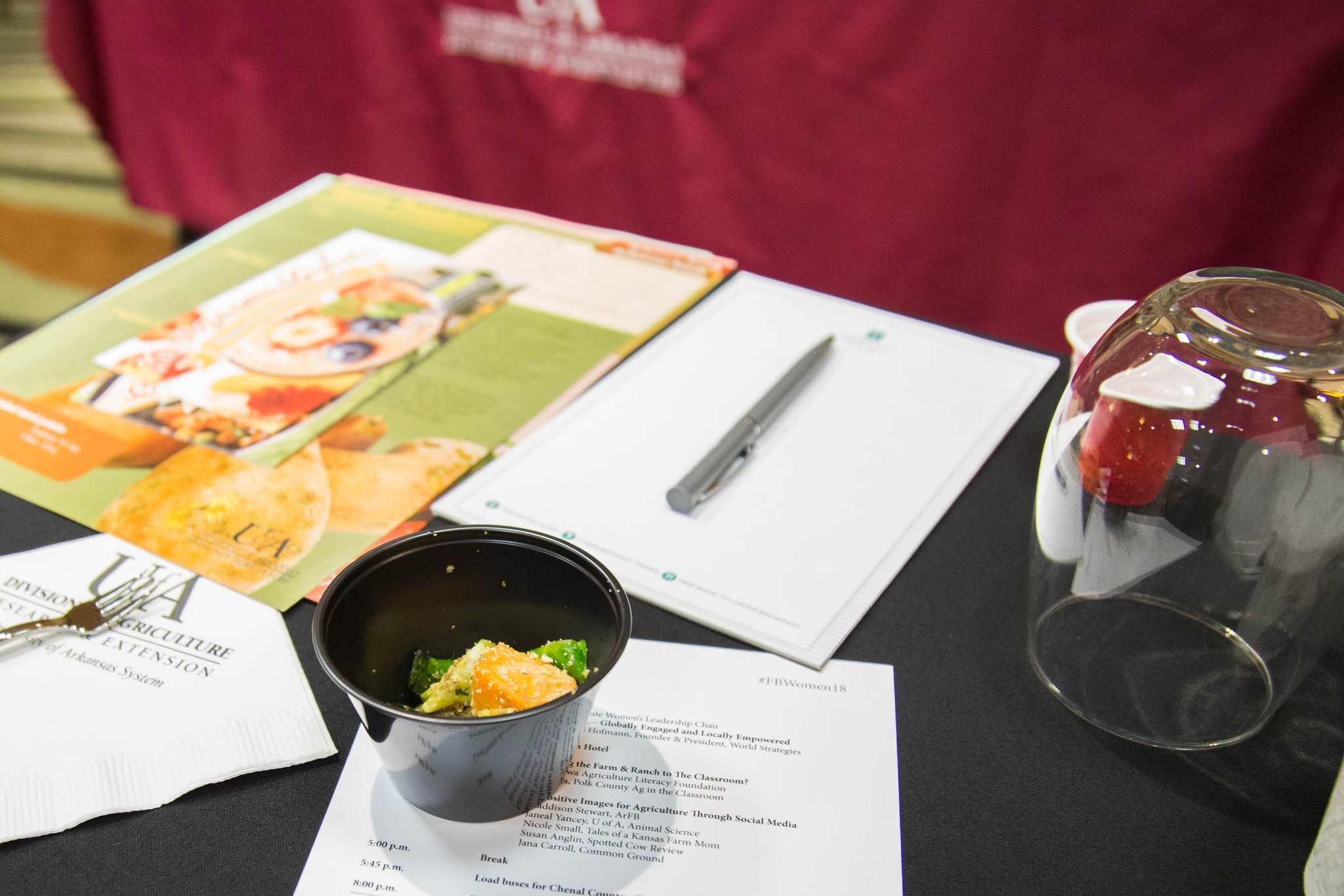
[409,639,588,700]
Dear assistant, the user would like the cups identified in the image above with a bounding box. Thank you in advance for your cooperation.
[310,524,633,825]
[1027,267,1344,754]
[1063,299,1230,506]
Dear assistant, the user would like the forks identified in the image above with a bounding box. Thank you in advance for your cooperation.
[0,573,159,642]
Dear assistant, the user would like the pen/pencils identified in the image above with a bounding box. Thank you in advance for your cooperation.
[667,337,832,512]
